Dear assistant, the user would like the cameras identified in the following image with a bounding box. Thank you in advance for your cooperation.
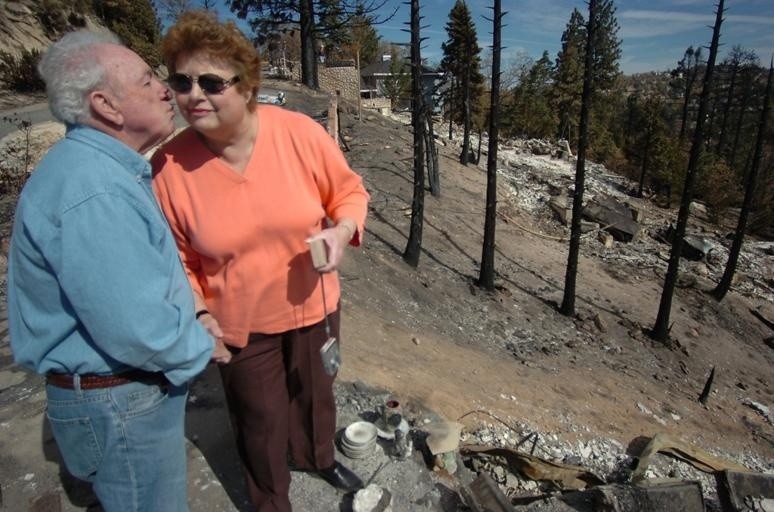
[320,338,340,376]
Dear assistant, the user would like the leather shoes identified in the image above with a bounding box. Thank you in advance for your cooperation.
[315,460,365,493]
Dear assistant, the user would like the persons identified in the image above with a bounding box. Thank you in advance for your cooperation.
[7,28,232,511]
[146,10,371,512]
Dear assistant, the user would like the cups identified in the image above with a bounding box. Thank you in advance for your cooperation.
[375,400,403,432]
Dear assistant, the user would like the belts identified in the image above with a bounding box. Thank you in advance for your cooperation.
[46,371,138,390]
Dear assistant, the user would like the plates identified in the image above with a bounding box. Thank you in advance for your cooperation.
[339,417,409,459]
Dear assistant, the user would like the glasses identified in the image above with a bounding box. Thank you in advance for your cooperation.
[167,73,241,95]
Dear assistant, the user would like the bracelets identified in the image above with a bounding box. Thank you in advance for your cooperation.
[195,309,210,320]
[335,222,353,243]
